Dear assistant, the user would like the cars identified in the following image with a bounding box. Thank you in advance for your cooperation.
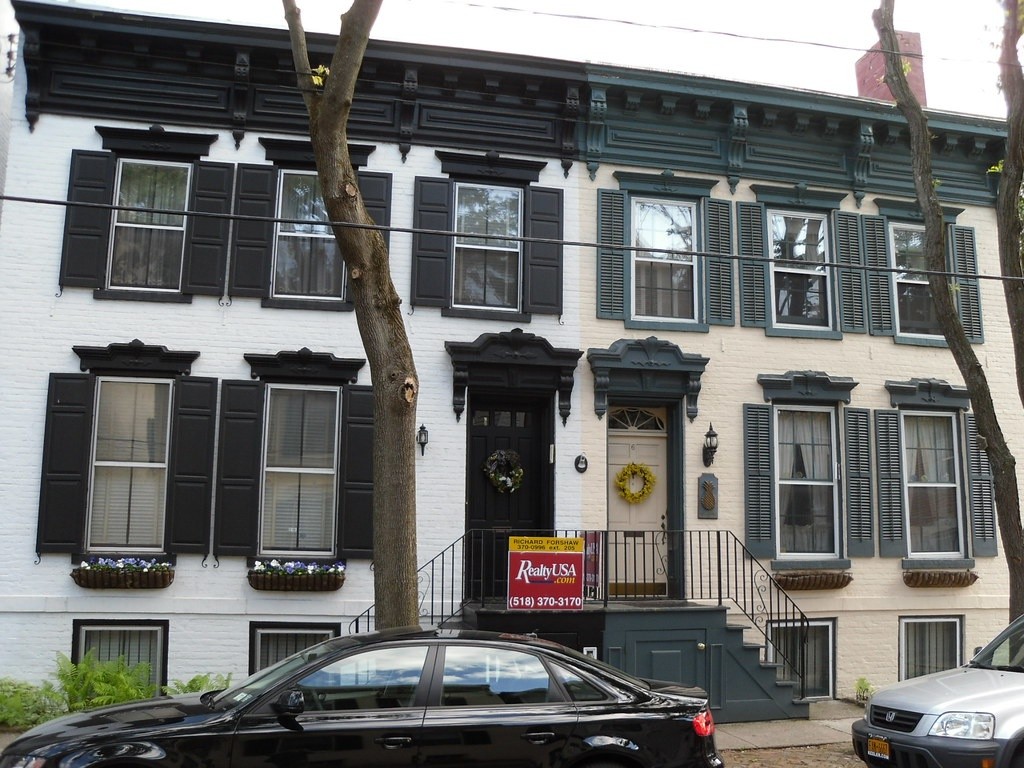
[850,612,1024,768]
[1,626,726,768]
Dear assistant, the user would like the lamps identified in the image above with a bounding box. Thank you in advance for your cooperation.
[416,423,428,456]
[703,422,718,467]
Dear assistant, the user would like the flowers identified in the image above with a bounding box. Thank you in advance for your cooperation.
[80,556,172,572]
[255,558,345,576]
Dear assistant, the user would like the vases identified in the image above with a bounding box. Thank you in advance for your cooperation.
[247,570,345,592]
[70,568,174,589]
